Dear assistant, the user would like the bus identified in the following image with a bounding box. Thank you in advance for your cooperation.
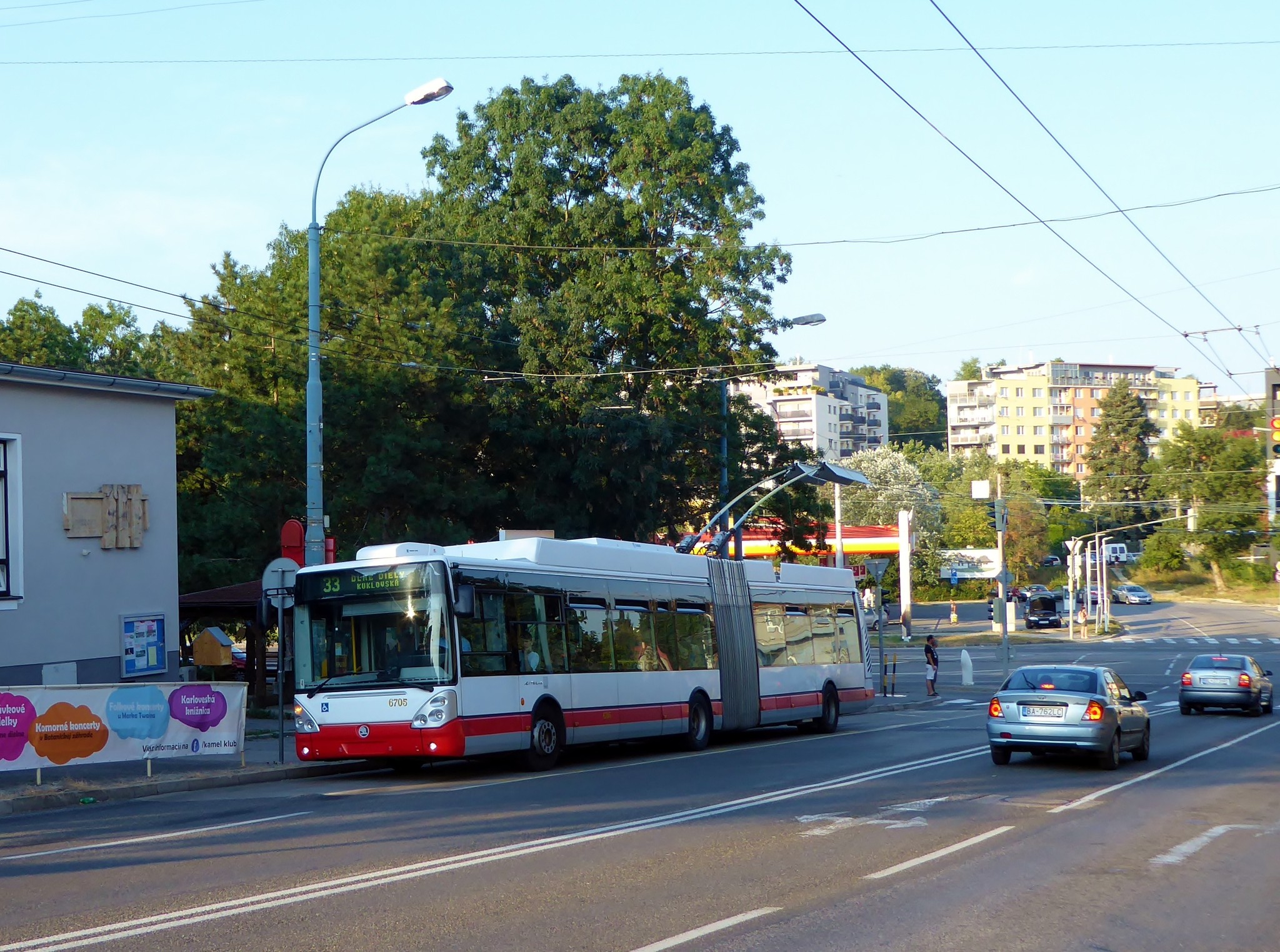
[263,460,877,771]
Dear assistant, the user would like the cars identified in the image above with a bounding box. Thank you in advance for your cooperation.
[1079,553,1144,564]
[988,583,1062,628]
[1044,555,1062,566]
[1178,653,1273,715]
[986,664,1151,771]
[1112,585,1152,605]
[231,647,257,682]
[1077,585,1110,605]
[864,608,889,631]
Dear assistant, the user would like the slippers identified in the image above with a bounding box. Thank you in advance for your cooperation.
[927,692,939,696]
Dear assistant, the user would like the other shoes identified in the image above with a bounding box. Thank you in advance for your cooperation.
[956,623,959,625]
[949,623,953,625]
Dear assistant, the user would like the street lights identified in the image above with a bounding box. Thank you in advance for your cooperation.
[717,312,827,560]
[1068,513,1199,637]
[303,75,456,565]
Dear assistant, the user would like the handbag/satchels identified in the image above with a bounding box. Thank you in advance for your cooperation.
[951,614,957,623]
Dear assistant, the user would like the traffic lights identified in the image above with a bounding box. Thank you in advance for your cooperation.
[1269,417,1280,456]
[985,500,1003,531]
[875,587,891,615]
[988,597,1004,623]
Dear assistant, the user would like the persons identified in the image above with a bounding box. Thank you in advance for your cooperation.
[924,634,941,697]
[1008,584,1021,608]
[418,614,681,672]
[949,598,959,626]
[1039,675,1052,685]
[1076,604,1089,639]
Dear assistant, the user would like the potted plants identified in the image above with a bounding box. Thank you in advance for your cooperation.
[773,385,826,396]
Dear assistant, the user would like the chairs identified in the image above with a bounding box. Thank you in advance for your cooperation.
[535,639,541,672]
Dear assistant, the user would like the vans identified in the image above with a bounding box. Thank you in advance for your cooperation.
[1100,543,1128,565]
